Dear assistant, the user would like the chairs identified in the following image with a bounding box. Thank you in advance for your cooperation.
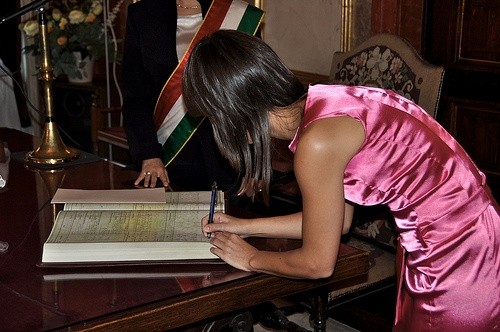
[298,32,447,332]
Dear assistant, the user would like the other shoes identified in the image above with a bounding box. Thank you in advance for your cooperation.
[259,309,290,330]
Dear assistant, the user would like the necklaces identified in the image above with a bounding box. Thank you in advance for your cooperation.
[176,3,201,9]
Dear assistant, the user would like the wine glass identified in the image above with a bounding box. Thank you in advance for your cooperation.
[0,139,10,253]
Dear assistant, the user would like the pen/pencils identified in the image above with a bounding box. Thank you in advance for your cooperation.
[208,181,217,239]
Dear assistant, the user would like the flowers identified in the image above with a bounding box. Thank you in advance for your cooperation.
[17,0,123,87]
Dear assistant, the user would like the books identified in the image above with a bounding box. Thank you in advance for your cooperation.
[37,189,228,268]
[42,262,230,282]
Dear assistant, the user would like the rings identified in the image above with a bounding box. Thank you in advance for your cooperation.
[145,171,152,175]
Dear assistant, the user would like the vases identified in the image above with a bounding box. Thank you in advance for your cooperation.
[67,52,95,83]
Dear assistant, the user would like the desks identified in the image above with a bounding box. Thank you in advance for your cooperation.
[0,147,373,332]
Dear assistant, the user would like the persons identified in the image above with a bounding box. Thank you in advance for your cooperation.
[124,0,262,199]
[182,28,500,332]
